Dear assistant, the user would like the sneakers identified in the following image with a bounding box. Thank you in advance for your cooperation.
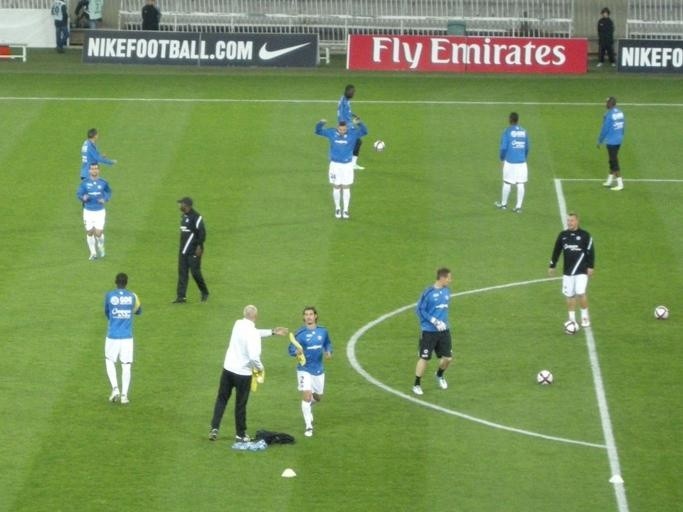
[121,397,130,404]
[603,182,613,187]
[439,377,448,389]
[171,298,186,304]
[512,207,521,214]
[235,434,252,443]
[596,62,603,67]
[610,186,624,191]
[208,428,218,440]
[611,62,616,67]
[88,249,106,260]
[494,202,508,209]
[200,293,208,301]
[304,426,313,437]
[581,317,591,328]
[333,165,364,218]
[109,389,120,403]
[412,385,424,395]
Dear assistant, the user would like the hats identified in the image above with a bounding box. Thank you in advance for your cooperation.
[178,197,192,206]
[601,8,609,15]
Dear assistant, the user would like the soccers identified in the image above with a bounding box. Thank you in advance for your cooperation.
[655,306,669,319]
[537,370,553,386]
[374,141,385,152]
[564,320,579,335]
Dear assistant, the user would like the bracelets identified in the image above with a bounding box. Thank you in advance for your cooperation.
[272,329,275,335]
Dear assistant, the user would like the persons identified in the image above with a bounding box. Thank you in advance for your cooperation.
[495,111,529,213]
[50,0,105,56]
[172,197,209,303]
[316,117,367,218]
[208,302,288,443]
[596,8,617,67]
[287,306,333,439]
[595,97,625,191]
[414,268,453,395]
[76,161,112,259]
[140,0,161,30]
[549,212,595,327]
[103,274,142,405]
[338,84,365,171]
[80,128,116,180]
